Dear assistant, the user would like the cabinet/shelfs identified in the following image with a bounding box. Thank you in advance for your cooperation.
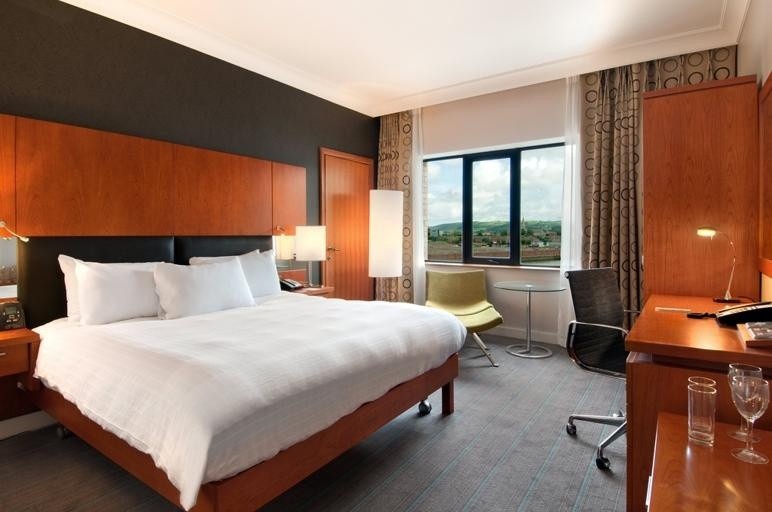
[643,75,759,297]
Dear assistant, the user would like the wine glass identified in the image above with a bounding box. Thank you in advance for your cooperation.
[732,376,771,465]
[726,363,763,444]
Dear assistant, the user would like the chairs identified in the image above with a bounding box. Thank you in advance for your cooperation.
[565,266,640,469]
[424,269,501,367]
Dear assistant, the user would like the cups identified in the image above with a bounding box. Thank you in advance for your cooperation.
[688,375,717,433]
[687,384,716,448]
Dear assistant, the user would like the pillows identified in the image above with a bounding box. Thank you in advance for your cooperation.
[58,248,282,325]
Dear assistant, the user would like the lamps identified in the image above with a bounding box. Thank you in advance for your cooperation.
[698,227,743,303]
[276,236,296,269]
[296,225,327,289]
[368,190,404,302]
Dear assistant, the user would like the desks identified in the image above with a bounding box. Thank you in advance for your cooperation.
[646,409,772,512]
[627,293,772,512]
[493,281,567,357]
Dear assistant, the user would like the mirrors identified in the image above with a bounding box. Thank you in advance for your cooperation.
[16,233,466,512]
[0,236,17,286]
[272,234,309,273]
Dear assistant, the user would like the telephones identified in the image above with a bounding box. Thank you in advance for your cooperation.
[715,300,772,326]
[280,278,303,291]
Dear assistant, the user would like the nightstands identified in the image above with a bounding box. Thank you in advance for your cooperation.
[0,328,41,393]
[295,284,334,298]
